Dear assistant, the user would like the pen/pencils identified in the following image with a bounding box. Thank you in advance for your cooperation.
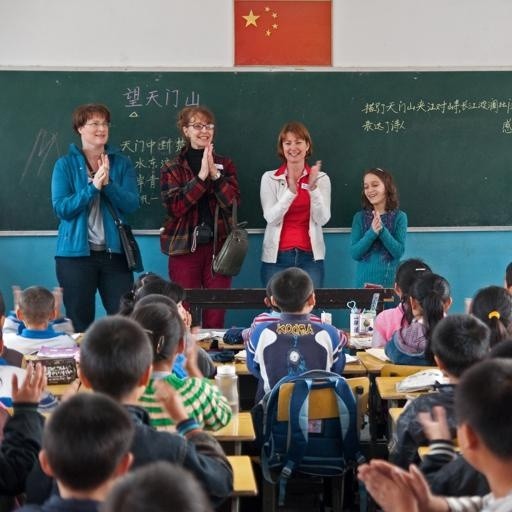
[347,301,371,314]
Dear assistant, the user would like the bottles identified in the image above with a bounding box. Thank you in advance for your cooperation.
[215,365,239,416]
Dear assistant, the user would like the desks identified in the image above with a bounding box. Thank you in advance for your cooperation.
[180,285,399,334]
[7,327,463,512]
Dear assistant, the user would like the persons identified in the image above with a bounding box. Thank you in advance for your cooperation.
[51,103,140,332]
[241,266,348,411]
[160,104,241,329]
[1,272,231,511]
[259,121,331,289]
[356,260,511,511]
[350,168,407,310]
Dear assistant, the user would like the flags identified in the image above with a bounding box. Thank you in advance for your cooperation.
[234,1,333,68]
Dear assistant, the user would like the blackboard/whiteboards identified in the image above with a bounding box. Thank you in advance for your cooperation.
[0,64,512,235]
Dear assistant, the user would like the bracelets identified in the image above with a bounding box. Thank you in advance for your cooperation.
[211,170,221,181]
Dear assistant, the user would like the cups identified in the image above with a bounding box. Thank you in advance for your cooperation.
[350,313,360,336]
[320,313,332,325]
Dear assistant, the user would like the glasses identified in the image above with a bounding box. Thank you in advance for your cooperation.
[84,121,112,127]
[187,123,215,130]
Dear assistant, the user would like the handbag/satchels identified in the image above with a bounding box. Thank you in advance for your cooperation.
[116,223,145,274]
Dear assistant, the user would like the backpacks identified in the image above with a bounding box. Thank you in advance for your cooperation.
[250,369,365,489]
[209,195,249,277]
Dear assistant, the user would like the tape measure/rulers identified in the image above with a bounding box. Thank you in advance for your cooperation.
[371,292,380,311]
